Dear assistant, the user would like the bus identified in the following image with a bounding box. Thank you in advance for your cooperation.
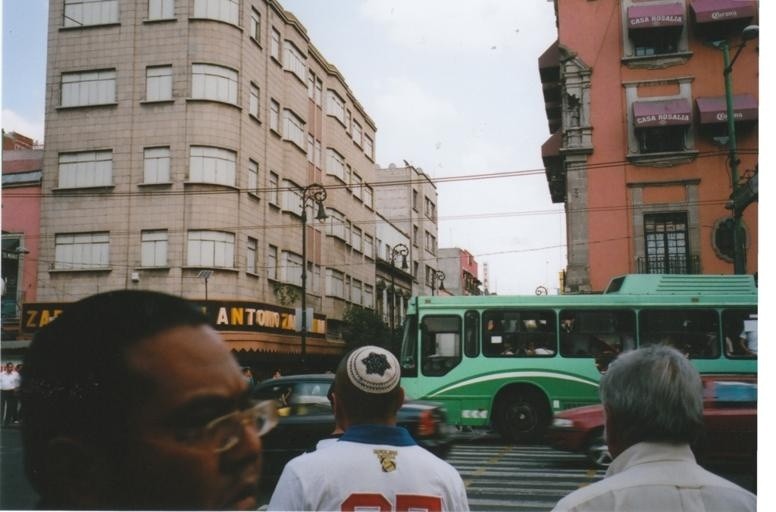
[397,270,756,446]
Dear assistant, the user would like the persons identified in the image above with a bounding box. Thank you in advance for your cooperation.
[22,289,262,511]
[242,368,252,382]
[273,368,288,406]
[549,345,757,512]
[261,343,471,511]
[0,362,23,431]
[485,309,758,357]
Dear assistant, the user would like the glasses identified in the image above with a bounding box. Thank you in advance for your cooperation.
[202,399,280,455]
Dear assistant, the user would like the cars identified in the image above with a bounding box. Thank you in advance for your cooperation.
[244,370,454,493]
[543,372,756,470]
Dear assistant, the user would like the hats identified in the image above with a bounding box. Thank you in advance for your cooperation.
[346,344,401,394]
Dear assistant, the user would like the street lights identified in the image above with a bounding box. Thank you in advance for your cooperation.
[429,270,447,297]
[299,181,329,370]
[709,23,756,273]
[387,243,408,353]
